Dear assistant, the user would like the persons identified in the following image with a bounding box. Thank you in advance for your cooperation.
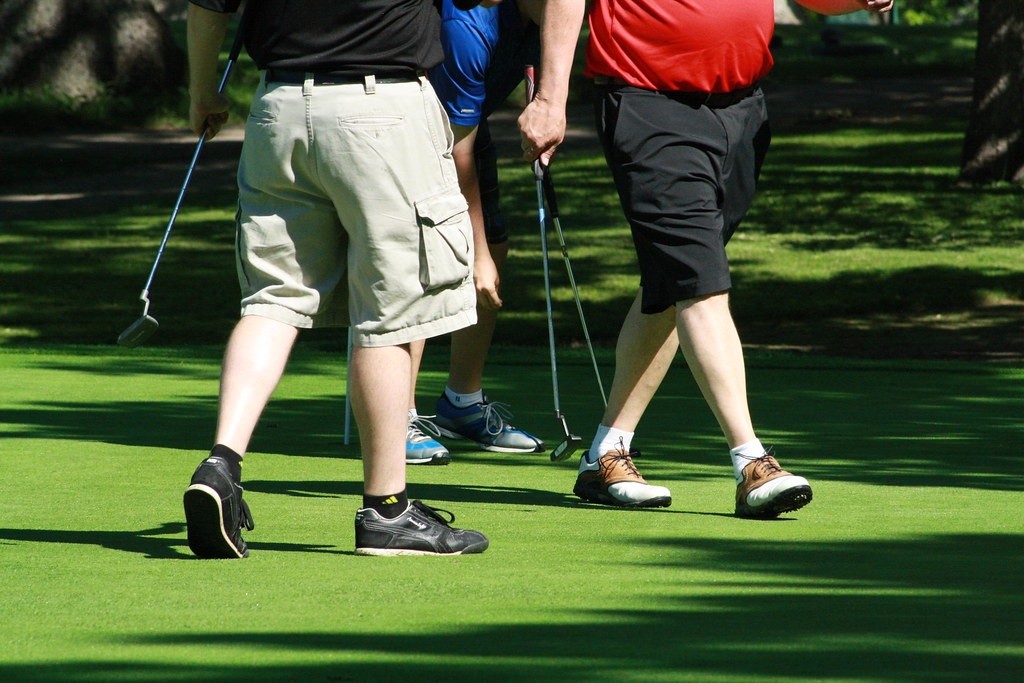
[406,0,559,465]
[183,0,488,560]
[518,0,894,519]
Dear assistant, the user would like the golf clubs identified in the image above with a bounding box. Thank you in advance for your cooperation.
[115,5,248,348]
[542,162,643,459]
[532,156,584,462]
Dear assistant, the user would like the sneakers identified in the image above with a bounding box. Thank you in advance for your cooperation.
[433,389,544,453]
[574,449,674,507]
[735,446,815,517]
[352,497,488,555]
[182,453,254,560]
[404,414,451,465]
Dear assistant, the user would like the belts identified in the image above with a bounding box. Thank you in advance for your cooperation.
[269,67,425,87]
